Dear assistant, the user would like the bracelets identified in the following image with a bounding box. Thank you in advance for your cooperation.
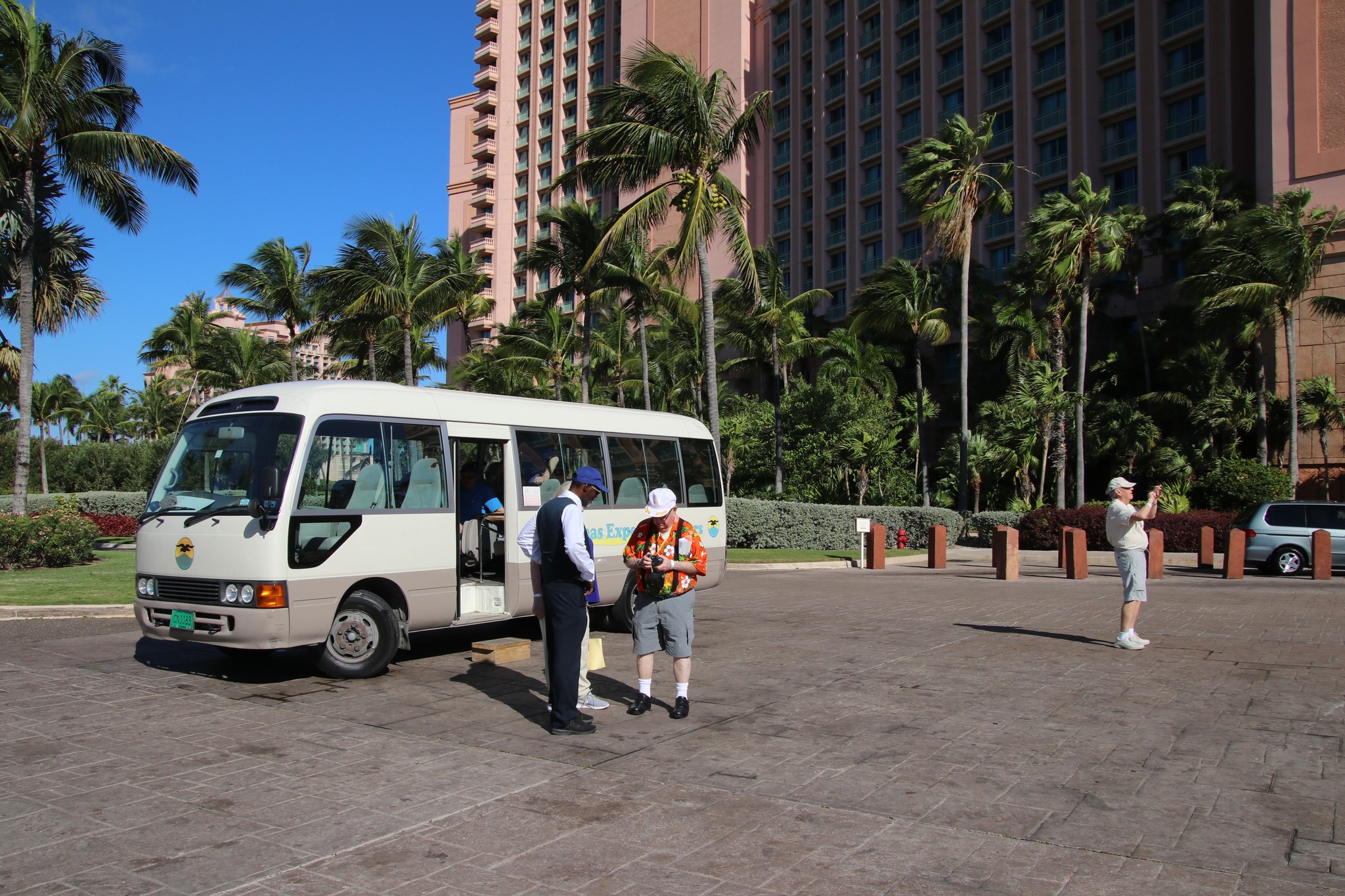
[670,559,674,570]
[1145,503,1151,507]
[533,594,543,597]
[1152,503,1157,506]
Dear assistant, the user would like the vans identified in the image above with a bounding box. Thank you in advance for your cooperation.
[1230,499,1345,577]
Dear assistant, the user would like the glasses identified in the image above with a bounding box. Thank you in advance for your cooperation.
[461,474,478,480]
[582,483,600,497]
[660,508,672,519]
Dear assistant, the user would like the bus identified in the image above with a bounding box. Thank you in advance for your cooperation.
[133,369,728,680]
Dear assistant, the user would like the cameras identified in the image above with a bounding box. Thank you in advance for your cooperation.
[1156,489,1161,495]
[648,555,663,566]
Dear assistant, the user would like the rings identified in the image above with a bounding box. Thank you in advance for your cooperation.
[539,479,541,481]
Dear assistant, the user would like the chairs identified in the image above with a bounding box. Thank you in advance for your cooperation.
[615,476,648,505]
[393,471,412,507]
[301,464,385,551]
[589,491,609,505]
[533,479,559,505]
[647,480,668,493]
[462,481,503,567]
[327,480,357,509]
[688,484,714,503]
[400,457,441,508]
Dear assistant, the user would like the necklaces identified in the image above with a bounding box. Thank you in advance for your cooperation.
[649,522,673,555]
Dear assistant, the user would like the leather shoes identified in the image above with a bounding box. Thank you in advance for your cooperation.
[670,696,689,718]
[628,693,651,714]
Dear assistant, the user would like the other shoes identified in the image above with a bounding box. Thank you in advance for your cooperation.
[464,555,477,566]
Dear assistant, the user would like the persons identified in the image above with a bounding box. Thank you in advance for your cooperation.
[1106,477,1162,651]
[531,480,610,710]
[623,487,707,719]
[516,431,559,486]
[517,466,610,734]
[459,462,505,577]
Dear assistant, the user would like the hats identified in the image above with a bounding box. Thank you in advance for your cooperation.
[572,466,610,493]
[1108,477,1137,493]
[644,488,676,518]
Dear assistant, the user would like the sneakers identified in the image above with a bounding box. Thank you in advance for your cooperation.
[574,708,594,723]
[576,688,609,709]
[1131,632,1150,645]
[546,703,552,711]
[552,716,597,735]
[1114,636,1144,650]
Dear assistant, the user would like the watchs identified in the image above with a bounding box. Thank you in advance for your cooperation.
[636,560,642,568]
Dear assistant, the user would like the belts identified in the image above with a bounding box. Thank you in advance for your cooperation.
[655,588,693,602]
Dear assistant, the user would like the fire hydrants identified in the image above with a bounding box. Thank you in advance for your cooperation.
[895,529,908,549]
